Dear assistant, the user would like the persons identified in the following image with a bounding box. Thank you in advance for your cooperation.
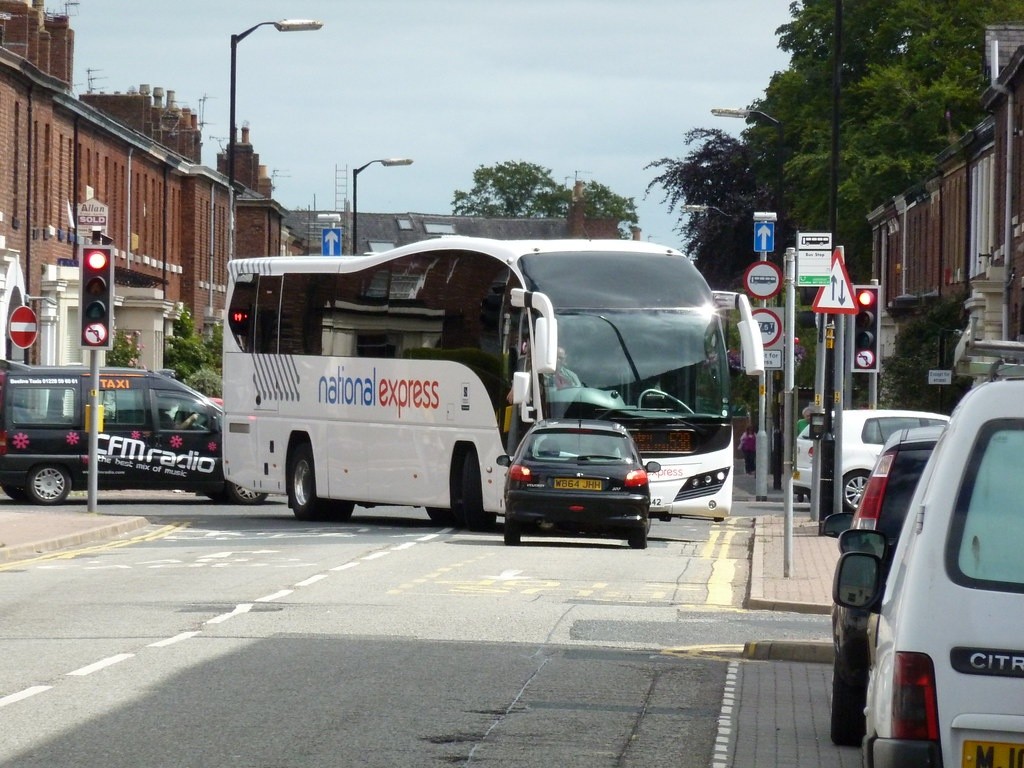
[742,426,757,473]
[798,407,816,436]
[507,347,581,404]
[774,426,783,462]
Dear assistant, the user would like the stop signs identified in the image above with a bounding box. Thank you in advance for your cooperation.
[10,306,39,350]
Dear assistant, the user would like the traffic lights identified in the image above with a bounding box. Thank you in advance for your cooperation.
[851,284,880,374]
[78,244,114,351]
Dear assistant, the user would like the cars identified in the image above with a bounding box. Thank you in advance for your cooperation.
[497,419,661,550]
[792,409,949,516]
[831,424,946,747]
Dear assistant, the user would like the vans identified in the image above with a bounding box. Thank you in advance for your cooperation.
[0,359,269,507]
[832,379,1024,768]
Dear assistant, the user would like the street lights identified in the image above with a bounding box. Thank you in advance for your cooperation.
[709,106,787,309]
[229,17,327,187]
[352,158,415,255]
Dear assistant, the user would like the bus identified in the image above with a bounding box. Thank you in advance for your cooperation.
[220,238,767,534]
